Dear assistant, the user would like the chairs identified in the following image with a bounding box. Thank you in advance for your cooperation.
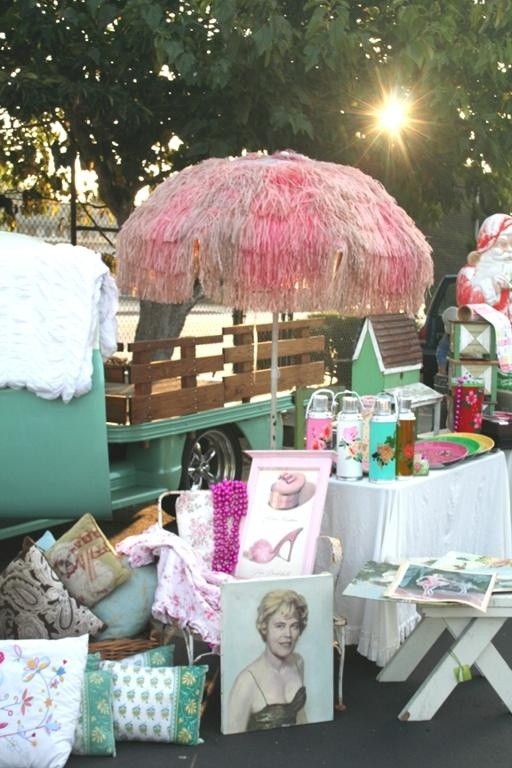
[158,491,349,713]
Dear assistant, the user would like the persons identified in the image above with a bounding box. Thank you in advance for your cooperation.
[225,586,311,734]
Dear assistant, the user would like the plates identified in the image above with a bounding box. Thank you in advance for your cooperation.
[414,432,496,466]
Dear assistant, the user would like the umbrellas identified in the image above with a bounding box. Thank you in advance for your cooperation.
[115,146,435,449]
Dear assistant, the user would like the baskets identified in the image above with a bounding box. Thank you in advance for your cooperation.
[88,639,159,660]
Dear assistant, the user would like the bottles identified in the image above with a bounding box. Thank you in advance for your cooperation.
[369,399,397,485]
[394,398,417,478]
[334,396,364,481]
[305,395,333,459]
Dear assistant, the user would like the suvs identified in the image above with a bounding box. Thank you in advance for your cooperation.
[415,271,461,388]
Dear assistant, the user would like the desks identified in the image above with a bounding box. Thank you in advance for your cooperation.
[316,435,506,648]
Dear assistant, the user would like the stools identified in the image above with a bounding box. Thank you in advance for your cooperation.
[377,577,512,722]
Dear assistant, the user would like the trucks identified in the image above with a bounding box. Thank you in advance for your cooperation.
[0,218,328,559]
